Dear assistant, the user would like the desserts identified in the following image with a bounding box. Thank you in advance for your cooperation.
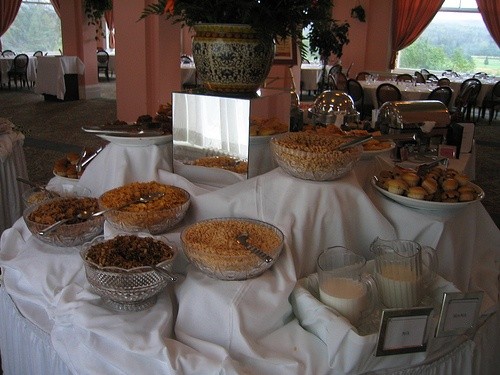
[377,164,479,203]
[53,152,93,179]
[250,115,288,137]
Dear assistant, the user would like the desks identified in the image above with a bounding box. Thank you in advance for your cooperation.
[0,55,38,88]
[358,81,455,110]
[96,50,115,77]
[430,72,500,114]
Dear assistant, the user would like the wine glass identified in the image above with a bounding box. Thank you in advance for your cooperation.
[412,75,418,90]
[391,75,398,84]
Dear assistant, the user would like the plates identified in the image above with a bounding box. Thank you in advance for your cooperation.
[372,169,484,213]
[95,134,172,147]
[250,132,288,145]
[53,170,80,182]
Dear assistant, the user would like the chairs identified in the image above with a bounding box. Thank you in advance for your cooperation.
[299,58,500,121]
[1,50,43,90]
[96,49,110,82]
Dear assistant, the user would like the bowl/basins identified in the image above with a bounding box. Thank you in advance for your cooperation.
[23,195,103,247]
[268,130,365,182]
[79,226,178,313]
[180,217,285,280]
[362,143,398,159]
[21,180,91,211]
[98,186,191,239]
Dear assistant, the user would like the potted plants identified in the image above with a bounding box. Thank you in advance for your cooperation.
[352,6,366,23]
[136,0,338,95]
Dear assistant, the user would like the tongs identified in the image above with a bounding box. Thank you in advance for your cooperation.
[82,118,164,136]
[415,156,451,176]
[334,134,373,152]
[76,143,102,172]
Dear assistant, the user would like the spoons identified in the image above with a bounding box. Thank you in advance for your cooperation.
[234,232,273,264]
[38,213,82,236]
[92,192,164,217]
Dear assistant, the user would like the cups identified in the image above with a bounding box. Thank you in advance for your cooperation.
[368,237,439,310]
[317,244,378,327]
